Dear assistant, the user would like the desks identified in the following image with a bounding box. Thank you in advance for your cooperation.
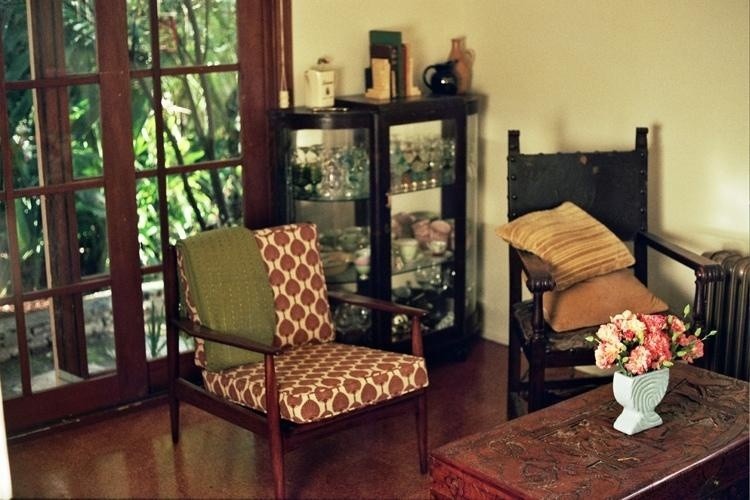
[430,360,747,500]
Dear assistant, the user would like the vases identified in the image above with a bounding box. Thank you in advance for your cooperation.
[609,365,670,435]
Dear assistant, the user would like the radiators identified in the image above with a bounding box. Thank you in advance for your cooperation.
[691,251,749,383]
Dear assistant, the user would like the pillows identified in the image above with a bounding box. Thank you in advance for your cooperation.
[490,202,669,333]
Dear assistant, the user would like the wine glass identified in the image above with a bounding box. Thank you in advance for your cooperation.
[332,303,373,341]
[413,262,462,295]
[386,134,454,180]
[294,142,369,198]
[354,258,369,280]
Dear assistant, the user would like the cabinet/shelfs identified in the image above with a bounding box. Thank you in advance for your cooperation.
[269,93,489,361]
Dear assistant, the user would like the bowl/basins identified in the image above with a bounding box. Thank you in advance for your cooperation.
[312,252,349,275]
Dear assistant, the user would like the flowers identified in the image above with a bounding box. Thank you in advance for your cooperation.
[583,305,716,376]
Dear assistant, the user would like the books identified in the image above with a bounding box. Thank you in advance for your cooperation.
[365,31,421,100]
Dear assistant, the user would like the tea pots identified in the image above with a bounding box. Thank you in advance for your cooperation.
[423,60,462,97]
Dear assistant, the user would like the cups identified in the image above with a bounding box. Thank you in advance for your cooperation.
[400,239,417,261]
[425,241,447,255]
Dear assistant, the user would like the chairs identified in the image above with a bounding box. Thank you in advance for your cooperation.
[164,223,430,499]
[506,126,724,419]
